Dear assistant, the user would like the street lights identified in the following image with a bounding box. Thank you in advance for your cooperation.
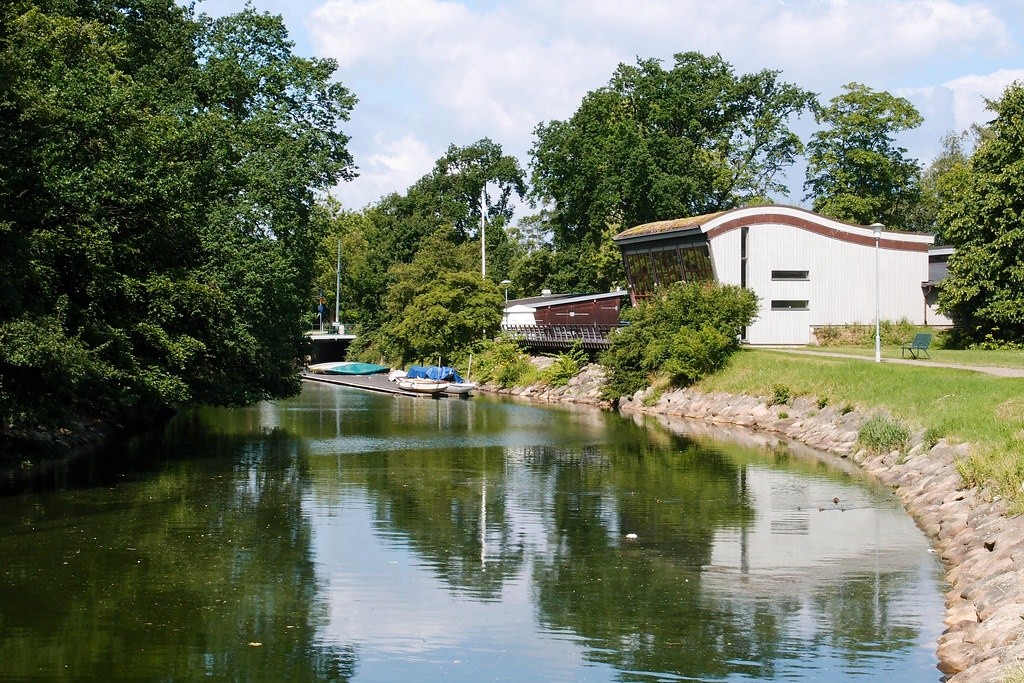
[871,222,883,363]
[502,280,511,330]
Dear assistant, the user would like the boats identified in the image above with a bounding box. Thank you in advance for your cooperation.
[308,353,477,394]
